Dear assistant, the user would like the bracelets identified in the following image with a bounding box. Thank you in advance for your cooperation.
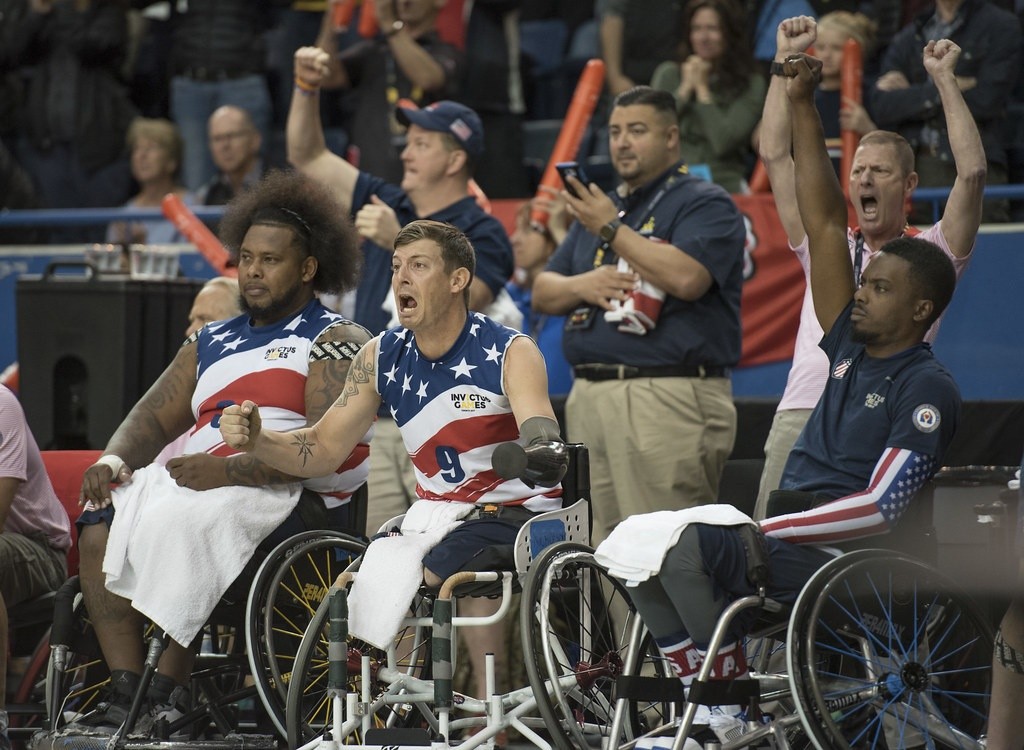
[770,60,790,77]
[295,76,320,92]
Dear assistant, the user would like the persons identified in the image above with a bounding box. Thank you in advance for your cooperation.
[218,220,565,748]
[533,86,740,732]
[1,0,1024,750]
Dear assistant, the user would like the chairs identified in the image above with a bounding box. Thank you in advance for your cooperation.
[2,450,123,743]
[477,0,910,188]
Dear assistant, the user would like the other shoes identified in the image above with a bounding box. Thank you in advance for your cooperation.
[59,670,148,734]
[128,675,198,742]
[462,713,508,749]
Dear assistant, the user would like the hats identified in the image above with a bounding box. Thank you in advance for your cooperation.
[395,97,486,158]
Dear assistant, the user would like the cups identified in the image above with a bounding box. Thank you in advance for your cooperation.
[85,243,180,281]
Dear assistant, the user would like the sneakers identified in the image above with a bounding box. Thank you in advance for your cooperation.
[634,704,773,750]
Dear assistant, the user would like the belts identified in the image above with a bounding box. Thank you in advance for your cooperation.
[575,363,734,379]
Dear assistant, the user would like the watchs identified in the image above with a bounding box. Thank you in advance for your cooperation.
[383,18,406,40]
[598,219,622,242]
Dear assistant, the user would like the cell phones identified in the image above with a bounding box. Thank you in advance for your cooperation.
[556,162,590,200]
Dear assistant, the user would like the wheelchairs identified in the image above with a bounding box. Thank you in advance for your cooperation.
[605,469,995,750]
[283,439,685,750]
[9,477,434,750]
